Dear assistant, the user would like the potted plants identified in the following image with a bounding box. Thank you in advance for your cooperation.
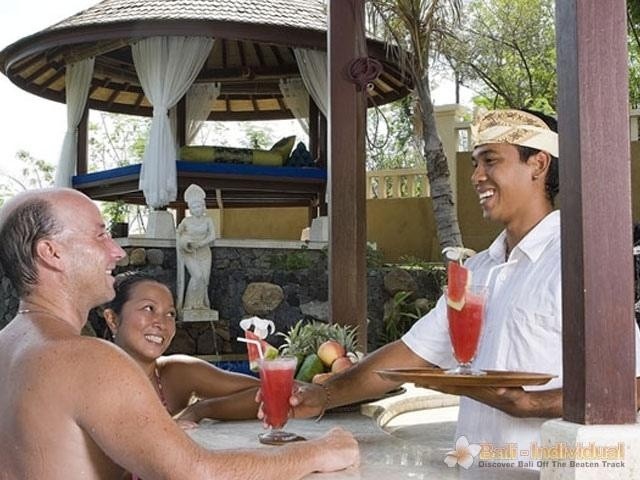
[102,199,129,238]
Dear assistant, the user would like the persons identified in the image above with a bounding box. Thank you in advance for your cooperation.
[173,184,219,321]
[1,189,360,480]
[255,106,640,475]
[102,269,261,480]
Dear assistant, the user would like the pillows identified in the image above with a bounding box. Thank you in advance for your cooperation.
[179,134,315,168]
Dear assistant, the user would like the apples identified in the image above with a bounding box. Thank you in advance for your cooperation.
[313,374,333,384]
[318,339,345,368]
[331,357,351,373]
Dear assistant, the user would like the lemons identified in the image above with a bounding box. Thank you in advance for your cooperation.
[294,353,323,382]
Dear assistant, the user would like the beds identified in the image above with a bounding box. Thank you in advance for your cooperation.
[71,160,325,226]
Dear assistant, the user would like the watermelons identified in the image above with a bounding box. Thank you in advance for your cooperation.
[447,261,468,311]
[245,329,279,374]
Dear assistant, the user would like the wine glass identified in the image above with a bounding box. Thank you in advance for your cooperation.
[440,283,490,376]
[255,356,301,443]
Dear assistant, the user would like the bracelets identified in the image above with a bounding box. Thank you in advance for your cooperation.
[317,382,331,421]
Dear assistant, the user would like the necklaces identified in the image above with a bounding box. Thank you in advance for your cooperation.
[17,309,35,315]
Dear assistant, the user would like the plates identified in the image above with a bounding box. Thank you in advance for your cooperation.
[371,363,559,391]
